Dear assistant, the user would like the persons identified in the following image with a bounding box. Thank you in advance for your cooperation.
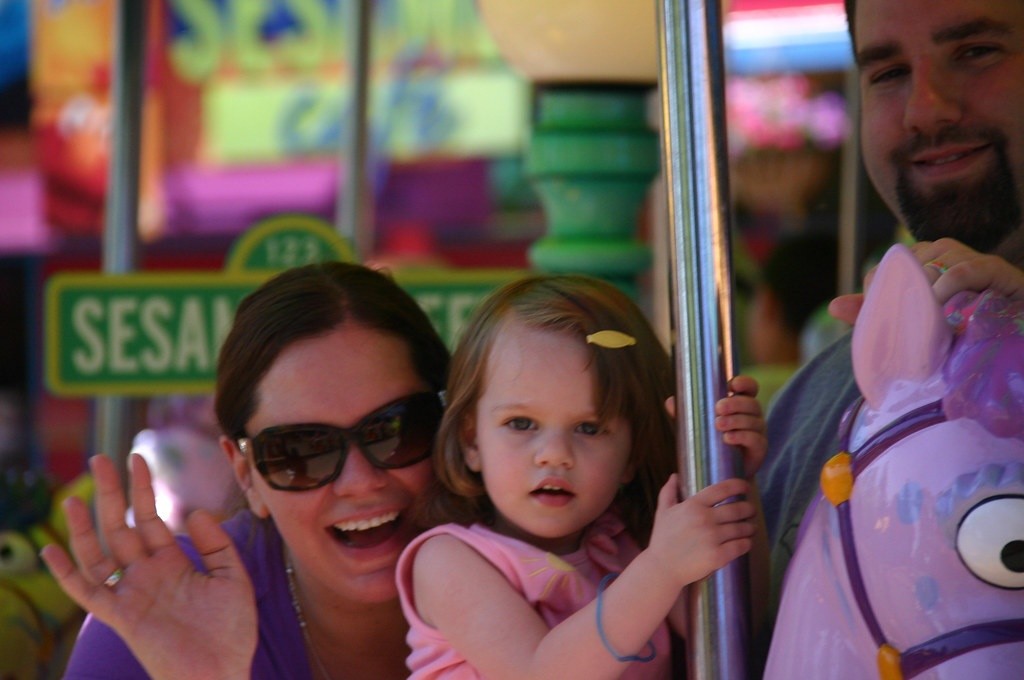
[759,0,1024,640]
[287,447,307,475]
[40,261,453,680]
[395,276,765,680]
[741,144,900,410]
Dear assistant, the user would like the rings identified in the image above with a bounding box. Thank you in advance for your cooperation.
[104,567,123,587]
[926,260,948,271]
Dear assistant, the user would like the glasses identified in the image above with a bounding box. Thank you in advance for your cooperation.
[237,390,448,494]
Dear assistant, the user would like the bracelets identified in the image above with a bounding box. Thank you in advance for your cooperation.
[596,573,657,662]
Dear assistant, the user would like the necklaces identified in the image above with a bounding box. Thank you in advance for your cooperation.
[286,556,329,680]
